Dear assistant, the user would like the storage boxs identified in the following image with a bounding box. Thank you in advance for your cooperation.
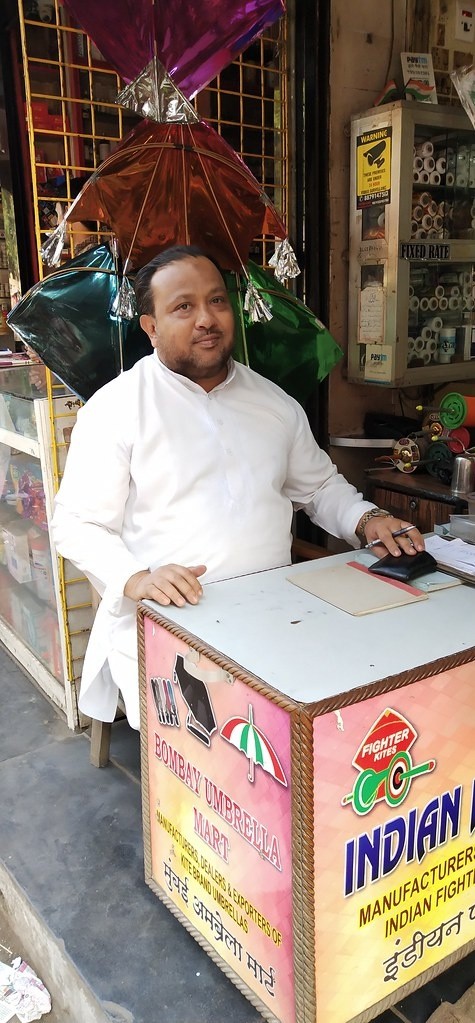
[0,521,64,682]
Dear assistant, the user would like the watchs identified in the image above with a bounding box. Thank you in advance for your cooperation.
[359,508,394,536]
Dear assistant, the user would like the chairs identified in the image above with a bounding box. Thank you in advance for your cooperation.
[88,689,128,769]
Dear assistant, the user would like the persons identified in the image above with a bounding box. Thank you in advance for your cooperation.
[25,176,112,391]
[45,246,423,729]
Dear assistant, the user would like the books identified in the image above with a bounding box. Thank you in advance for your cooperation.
[354,549,466,593]
[405,534,475,584]
[287,560,428,616]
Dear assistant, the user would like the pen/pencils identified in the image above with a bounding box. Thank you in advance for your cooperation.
[365,524,417,549]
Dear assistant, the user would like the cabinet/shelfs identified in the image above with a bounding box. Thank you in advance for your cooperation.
[0,362,124,736]
[137,460,475,1023]
[348,100,475,386]
